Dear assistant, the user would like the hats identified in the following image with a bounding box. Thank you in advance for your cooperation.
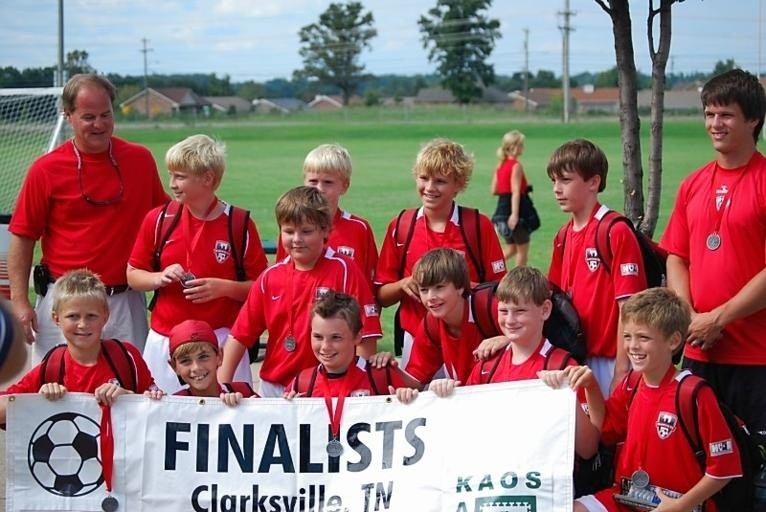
[170,320,218,357]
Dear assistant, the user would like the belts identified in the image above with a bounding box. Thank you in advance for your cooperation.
[104,285,130,296]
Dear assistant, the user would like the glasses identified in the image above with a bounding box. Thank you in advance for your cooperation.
[77,165,124,206]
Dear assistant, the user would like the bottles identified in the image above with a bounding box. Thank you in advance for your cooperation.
[625,484,661,506]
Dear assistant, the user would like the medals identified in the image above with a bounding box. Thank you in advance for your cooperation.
[284,334,296,352]
[631,469,650,489]
[326,439,344,459]
[706,231,721,251]
[180,271,196,289]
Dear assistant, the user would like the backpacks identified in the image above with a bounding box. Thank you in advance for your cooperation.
[558,210,664,289]
[477,346,616,499]
[425,280,587,366]
[626,369,761,512]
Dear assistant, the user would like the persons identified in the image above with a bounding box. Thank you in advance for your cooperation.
[491,129,541,267]
[143,319,261,407]
[126,133,270,396]
[216,184,384,407]
[367,246,512,386]
[0,294,29,389]
[428,266,601,502]
[560,287,744,512]
[0,268,155,433]
[371,138,509,392]
[273,143,382,316]
[657,69,766,512]
[282,289,419,404]
[6,75,173,369]
[544,138,646,402]
